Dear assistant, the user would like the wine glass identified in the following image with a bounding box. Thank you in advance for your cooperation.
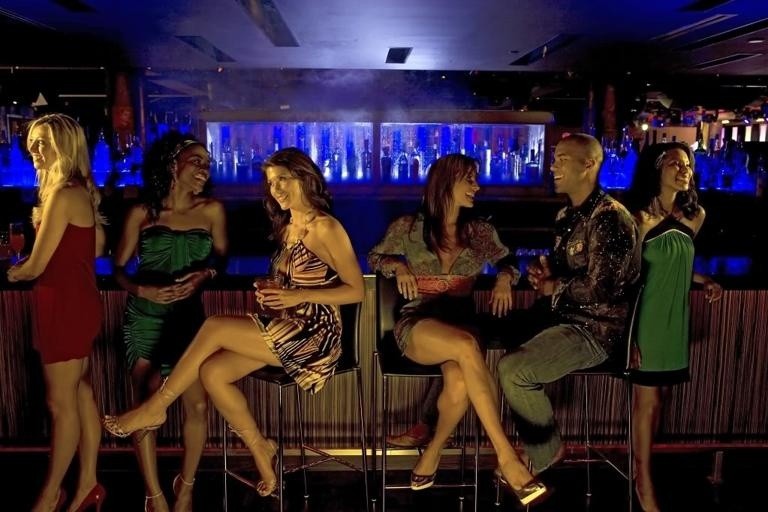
[9,223,25,260]
[86,111,192,173]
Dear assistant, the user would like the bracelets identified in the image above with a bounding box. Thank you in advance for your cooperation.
[209,268,217,278]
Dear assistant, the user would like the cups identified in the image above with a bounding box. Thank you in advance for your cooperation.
[255,276,284,316]
[0,232,14,260]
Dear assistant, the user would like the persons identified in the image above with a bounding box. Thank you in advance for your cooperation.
[368,153,548,505]
[109,130,230,512]
[387,133,638,484]
[2,112,110,511]
[629,142,724,512]
[99,147,367,505]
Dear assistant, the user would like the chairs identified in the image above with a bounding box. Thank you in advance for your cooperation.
[222,303,371,511]
[373,268,484,511]
[494,281,643,512]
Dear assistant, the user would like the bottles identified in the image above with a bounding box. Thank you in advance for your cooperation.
[643,129,767,198]
[209,136,544,186]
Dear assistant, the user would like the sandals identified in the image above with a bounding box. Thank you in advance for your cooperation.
[144,491,165,512]
[170,473,197,503]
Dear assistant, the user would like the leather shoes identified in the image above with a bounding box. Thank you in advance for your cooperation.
[498,457,548,507]
[378,423,435,449]
[493,445,564,480]
[410,444,444,491]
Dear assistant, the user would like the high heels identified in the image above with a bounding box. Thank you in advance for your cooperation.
[51,486,69,512]
[256,439,282,498]
[99,415,162,443]
[64,482,108,512]
